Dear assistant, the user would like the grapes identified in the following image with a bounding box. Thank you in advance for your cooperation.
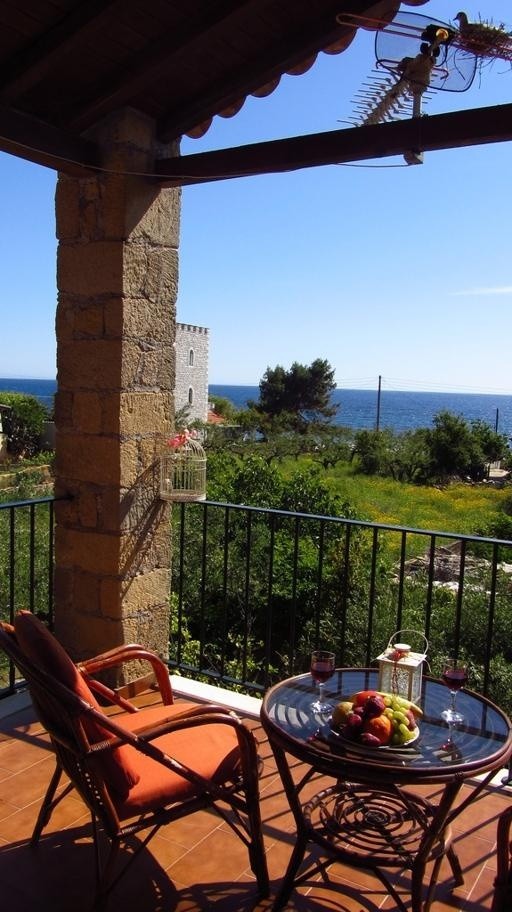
[383,695,415,743]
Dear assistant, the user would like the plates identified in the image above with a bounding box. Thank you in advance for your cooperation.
[326,714,420,750]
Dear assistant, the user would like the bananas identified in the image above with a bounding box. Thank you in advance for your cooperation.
[377,691,424,717]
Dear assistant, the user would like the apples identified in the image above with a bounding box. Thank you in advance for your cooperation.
[406,710,417,731]
[340,714,363,739]
[354,705,366,716]
[366,695,385,715]
[359,732,381,746]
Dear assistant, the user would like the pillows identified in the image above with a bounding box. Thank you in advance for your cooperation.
[13,610,139,791]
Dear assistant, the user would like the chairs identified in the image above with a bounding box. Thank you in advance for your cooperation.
[0,622,270,911]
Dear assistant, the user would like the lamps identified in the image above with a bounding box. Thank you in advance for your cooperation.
[377,630,431,704]
[491,805,512,912]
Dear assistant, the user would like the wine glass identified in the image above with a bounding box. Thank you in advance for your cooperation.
[439,658,468,723]
[308,650,337,715]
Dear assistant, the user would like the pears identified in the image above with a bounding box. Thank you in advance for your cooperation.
[330,702,354,726]
